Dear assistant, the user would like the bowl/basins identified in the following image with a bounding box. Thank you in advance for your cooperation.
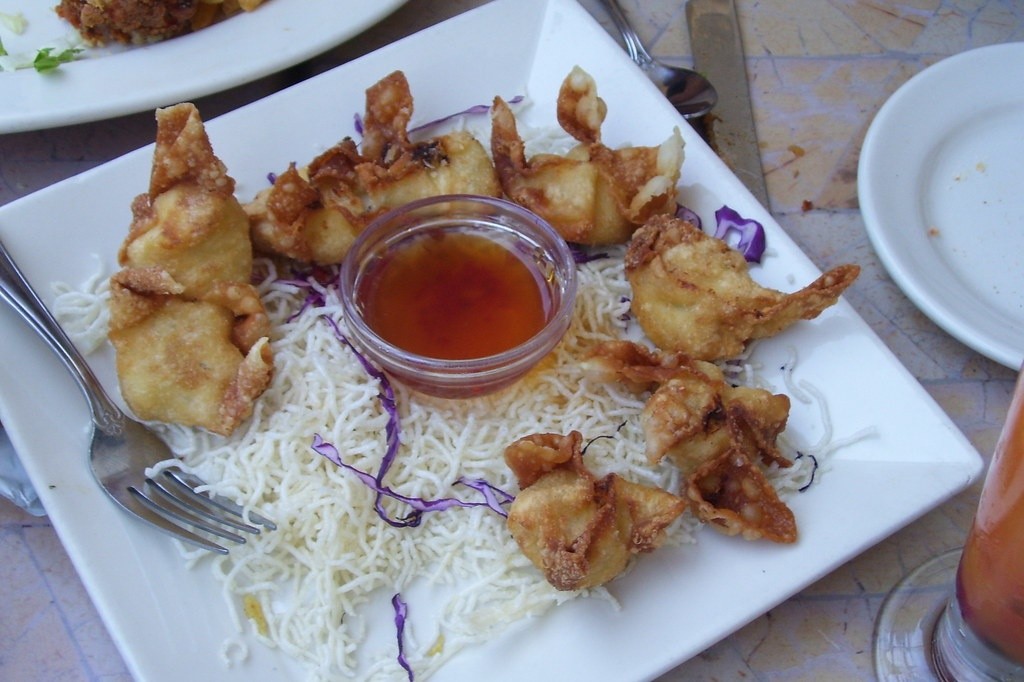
[339,192,579,401]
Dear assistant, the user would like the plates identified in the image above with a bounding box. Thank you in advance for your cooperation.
[0,0,411,134]
[0,0,988,682]
[855,39,1024,374]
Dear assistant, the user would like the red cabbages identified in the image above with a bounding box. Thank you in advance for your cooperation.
[268,95,821,681]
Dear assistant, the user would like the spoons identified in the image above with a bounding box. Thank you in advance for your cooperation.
[594,0,719,119]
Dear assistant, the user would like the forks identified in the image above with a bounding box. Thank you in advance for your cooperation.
[1,240,278,555]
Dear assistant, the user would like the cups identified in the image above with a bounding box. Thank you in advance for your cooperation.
[869,358,1024,682]
